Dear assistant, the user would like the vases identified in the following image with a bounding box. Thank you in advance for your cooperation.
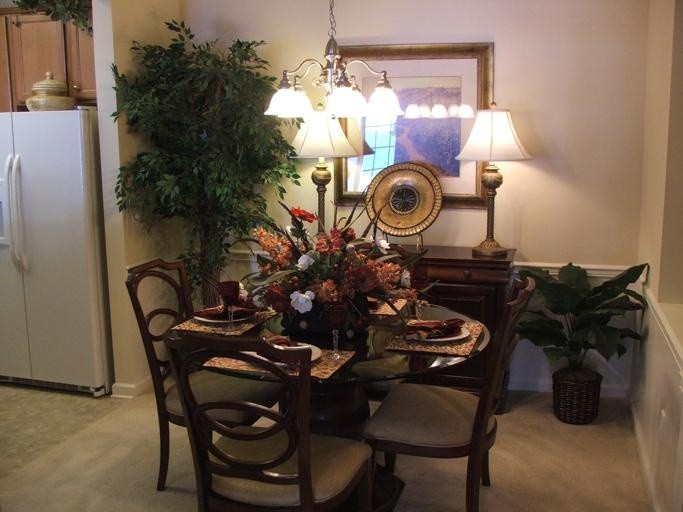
[311,298,361,341]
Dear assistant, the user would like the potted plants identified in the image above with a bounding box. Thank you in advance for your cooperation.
[511,260,651,425]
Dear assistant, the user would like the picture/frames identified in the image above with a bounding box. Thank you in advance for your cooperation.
[331,41,495,210]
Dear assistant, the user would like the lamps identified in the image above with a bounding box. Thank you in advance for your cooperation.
[286,117,359,239]
[454,108,534,258]
[403,88,475,122]
[260,0,405,124]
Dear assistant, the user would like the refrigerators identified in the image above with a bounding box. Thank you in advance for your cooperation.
[0,103,119,399]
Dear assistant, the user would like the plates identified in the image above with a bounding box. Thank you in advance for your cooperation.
[192,304,471,366]
[365,163,444,237]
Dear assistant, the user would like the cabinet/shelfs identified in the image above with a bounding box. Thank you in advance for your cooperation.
[357,245,517,415]
[10,8,96,107]
[0,11,18,113]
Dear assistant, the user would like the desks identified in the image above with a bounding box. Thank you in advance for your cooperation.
[167,299,493,511]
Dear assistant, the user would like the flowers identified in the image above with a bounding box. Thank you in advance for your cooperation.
[231,185,441,339]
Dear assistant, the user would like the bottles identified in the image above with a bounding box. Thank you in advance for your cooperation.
[25,71,76,111]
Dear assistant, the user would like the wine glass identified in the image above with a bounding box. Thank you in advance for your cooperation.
[215,279,240,332]
[322,298,350,361]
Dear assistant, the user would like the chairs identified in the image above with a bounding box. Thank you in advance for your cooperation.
[162,335,374,511]
[361,276,536,511]
[339,241,432,475]
[125,258,294,491]
